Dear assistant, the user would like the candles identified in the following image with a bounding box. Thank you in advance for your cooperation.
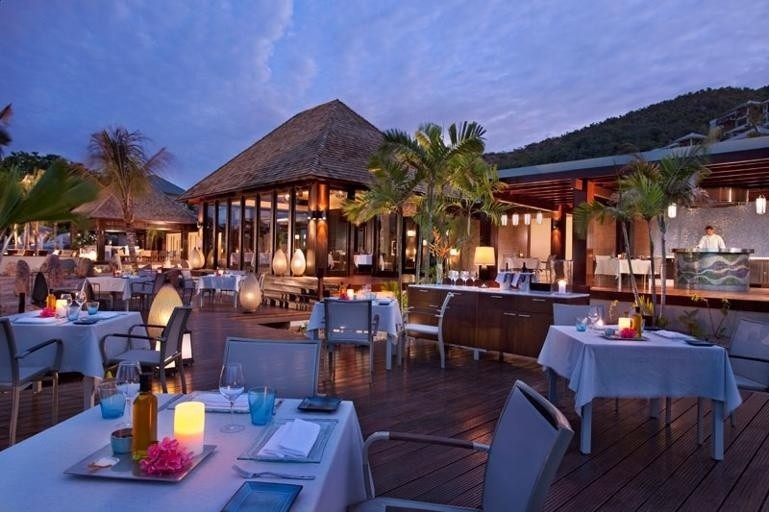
[558,280,568,294]
[617,316,631,333]
[174,400,205,457]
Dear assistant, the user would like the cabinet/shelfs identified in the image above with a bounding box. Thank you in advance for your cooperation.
[478,293,591,358]
[408,287,477,351]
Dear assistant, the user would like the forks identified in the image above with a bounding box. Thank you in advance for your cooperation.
[231,464,315,484]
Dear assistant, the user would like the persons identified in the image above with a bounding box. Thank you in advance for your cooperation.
[110,248,123,273]
[694,224,726,248]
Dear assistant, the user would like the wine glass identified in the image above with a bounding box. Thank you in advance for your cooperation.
[75,291,88,315]
[114,359,142,430]
[215,362,247,436]
[447,270,479,287]
[586,301,600,334]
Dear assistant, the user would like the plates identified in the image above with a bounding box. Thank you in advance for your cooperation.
[684,340,715,347]
[236,416,338,465]
[75,319,98,325]
[603,332,649,341]
[298,395,341,414]
[220,481,304,512]
[63,440,218,485]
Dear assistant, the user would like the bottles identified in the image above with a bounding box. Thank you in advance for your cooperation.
[630,306,643,337]
[339,281,346,297]
[46,289,55,309]
[131,371,159,461]
[503,263,528,273]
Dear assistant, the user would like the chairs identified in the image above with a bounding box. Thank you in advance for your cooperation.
[661,317,769,446]
[349,377,576,511]
[539,253,557,282]
[551,300,619,416]
[113,248,170,263]
[322,298,379,375]
[395,290,455,370]
[0,316,65,445]
[48,265,265,317]
[99,303,194,395]
[219,334,323,400]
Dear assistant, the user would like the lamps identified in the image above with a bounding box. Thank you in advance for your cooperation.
[270,249,288,277]
[500,204,543,227]
[188,247,207,269]
[290,248,307,276]
[755,192,766,215]
[474,246,496,288]
[146,285,184,337]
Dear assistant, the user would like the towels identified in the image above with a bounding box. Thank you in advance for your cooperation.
[258,417,321,459]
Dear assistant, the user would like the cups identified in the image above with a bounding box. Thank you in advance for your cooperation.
[60,294,73,306]
[248,384,276,425]
[573,316,587,330]
[67,308,80,322]
[111,428,132,454]
[96,381,129,419]
[84,301,100,315]
